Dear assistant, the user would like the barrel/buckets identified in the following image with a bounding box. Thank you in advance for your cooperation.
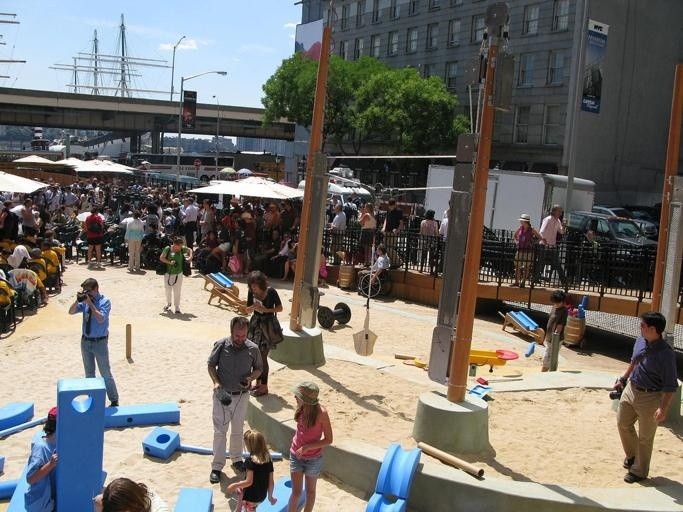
[564,314,586,346]
[338,264,355,289]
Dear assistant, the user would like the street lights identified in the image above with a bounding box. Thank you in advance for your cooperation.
[174,70,229,195]
[170,34,187,99]
[209,91,220,181]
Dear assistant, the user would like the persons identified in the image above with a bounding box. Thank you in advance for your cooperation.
[92,478,152,512]
[510,214,547,288]
[207,316,264,483]
[1,174,301,305]
[533,204,573,285]
[68,278,119,407]
[319,196,450,296]
[245,270,283,397]
[227,430,277,512]
[288,381,333,512]
[612,311,679,483]
[541,290,568,372]
[160,237,193,314]
[23,406,57,512]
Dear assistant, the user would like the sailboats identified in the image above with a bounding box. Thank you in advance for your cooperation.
[27,8,183,160]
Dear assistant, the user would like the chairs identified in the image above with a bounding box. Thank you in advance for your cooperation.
[0,218,112,337]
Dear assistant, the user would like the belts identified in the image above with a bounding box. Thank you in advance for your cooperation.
[83,336,106,341]
[226,390,247,395]
[636,387,662,392]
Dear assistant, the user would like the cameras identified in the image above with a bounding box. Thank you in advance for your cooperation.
[172,259,177,267]
[76,290,90,302]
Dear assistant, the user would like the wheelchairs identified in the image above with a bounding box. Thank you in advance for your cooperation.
[358,271,393,299]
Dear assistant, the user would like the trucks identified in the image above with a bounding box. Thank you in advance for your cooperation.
[420,160,657,292]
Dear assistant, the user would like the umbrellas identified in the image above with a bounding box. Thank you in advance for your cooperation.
[219,167,236,173]
[236,168,252,174]
[11,153,138,174]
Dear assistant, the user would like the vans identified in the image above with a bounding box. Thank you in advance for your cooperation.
[297,178,373,215]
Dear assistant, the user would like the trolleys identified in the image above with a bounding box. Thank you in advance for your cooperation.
[556,306,594,353]
[495,306,546,346]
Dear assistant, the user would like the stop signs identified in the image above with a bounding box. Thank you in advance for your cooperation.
[193,158,202,168]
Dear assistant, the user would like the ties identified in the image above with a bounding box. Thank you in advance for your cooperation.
[85,300,93,335]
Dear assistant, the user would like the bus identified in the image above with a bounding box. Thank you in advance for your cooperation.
[145,169,199,185]
[125,150,237,183]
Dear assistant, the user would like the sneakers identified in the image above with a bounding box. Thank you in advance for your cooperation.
[164,304,180,313]
[210,470,220,483]
[232,460,245,472]
[87,261,103,270]
[128,266,143,273]
[623,455,647,484]
[249,384,268,397]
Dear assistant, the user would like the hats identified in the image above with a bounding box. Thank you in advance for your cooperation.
[516,214,532,222]
[48,406,57,421]
[289,381,320,406]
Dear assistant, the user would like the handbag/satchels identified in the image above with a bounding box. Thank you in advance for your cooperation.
[182,259,191,277]
[258,315,284,344]
[155,262,167,275]
[87,217,102,231]
